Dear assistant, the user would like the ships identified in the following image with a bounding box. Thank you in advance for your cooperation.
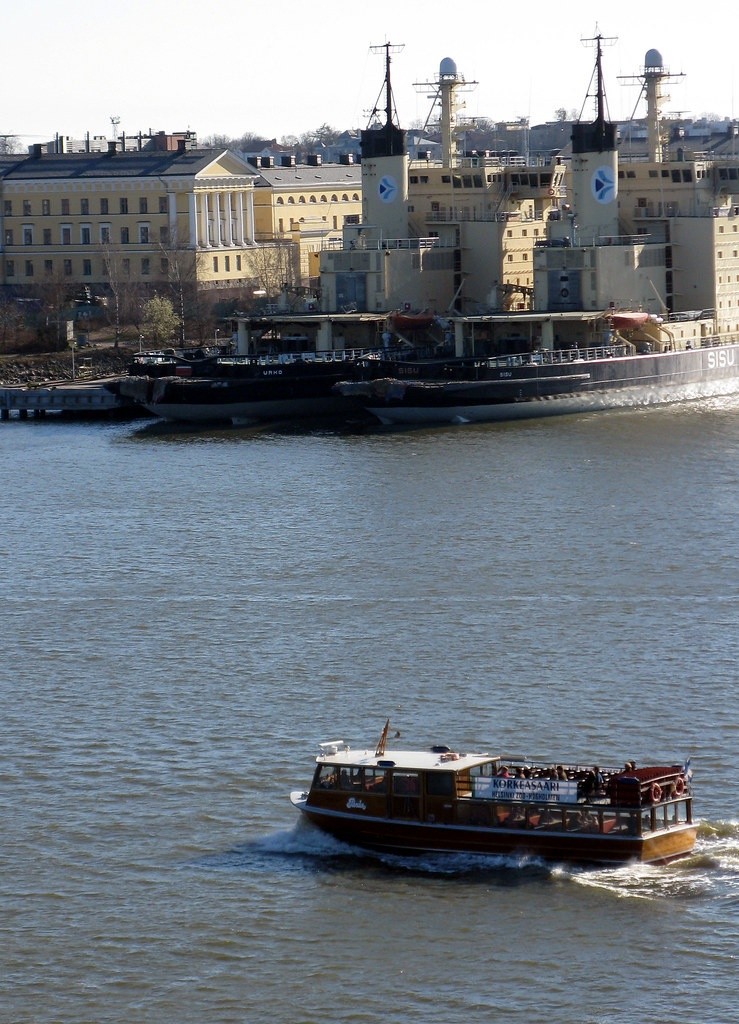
[103,310,738,426]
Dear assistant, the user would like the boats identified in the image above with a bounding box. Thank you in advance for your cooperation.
[289,716,701,874]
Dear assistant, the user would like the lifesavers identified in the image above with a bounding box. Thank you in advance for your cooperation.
[650,783,662,803]
[672,777,685,797]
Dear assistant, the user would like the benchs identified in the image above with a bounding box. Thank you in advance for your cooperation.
[497,813,619,834]
[530,766,616,798]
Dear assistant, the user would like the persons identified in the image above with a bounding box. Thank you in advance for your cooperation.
[538,809,554,826]
[623,763,633,772]
[568,810,599,834]
[497,765,510,779]
[550,766,567,781]
[337,769,350,786]
[514,765,531,779]
[502,811,520,828]
[580,767,604,805]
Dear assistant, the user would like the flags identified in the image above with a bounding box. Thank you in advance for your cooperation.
[682,756,693,783]
